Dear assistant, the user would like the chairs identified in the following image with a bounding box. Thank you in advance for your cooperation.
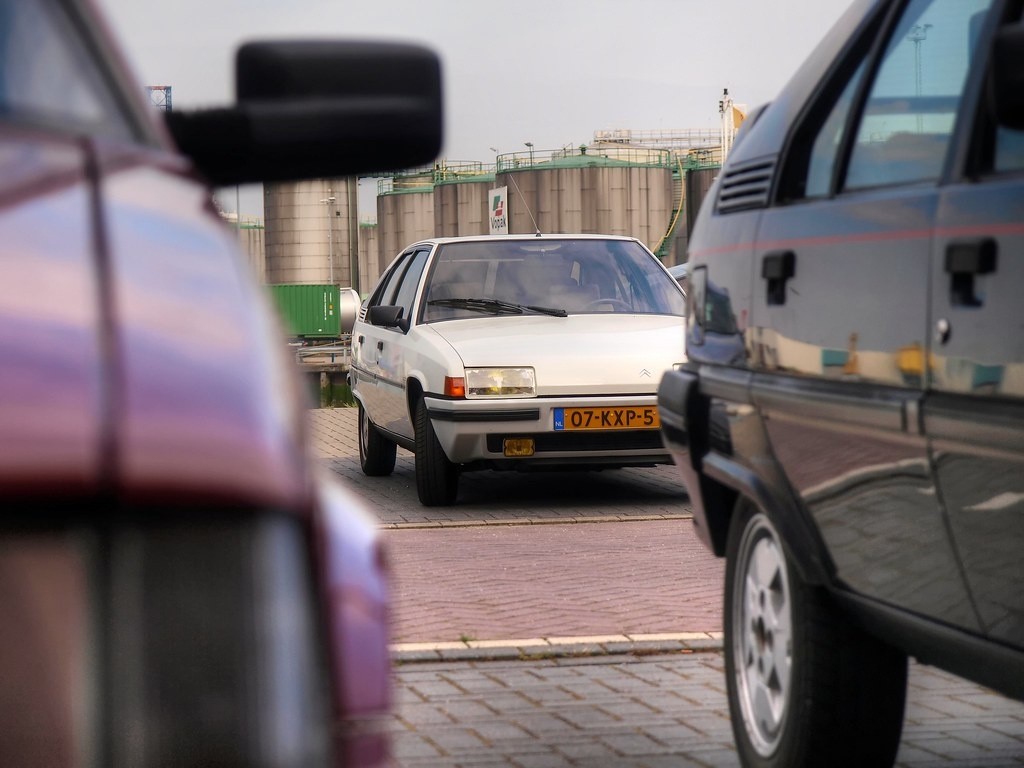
[430,280,488,317]
[516,252,580,296]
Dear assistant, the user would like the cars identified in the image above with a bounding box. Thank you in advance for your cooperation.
[346,234,691,506]
[0,0,447,768]
[655,0,1023,768]
